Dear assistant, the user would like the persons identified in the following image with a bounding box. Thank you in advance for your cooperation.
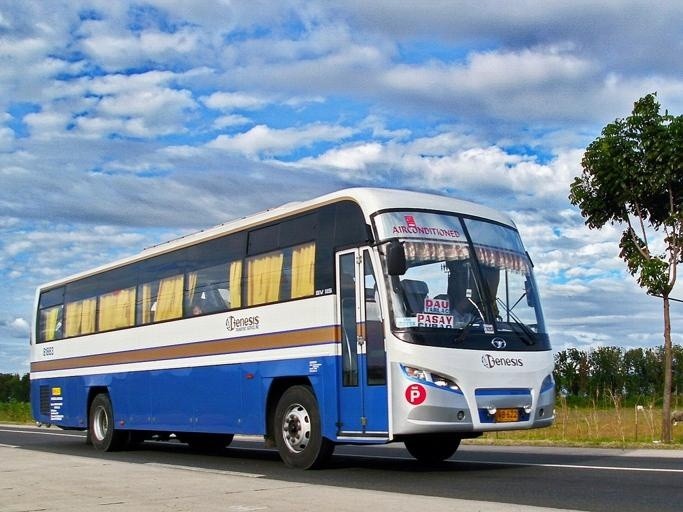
[191,303,206,315]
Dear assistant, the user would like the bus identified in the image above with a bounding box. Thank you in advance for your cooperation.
[29,186,557,470]
